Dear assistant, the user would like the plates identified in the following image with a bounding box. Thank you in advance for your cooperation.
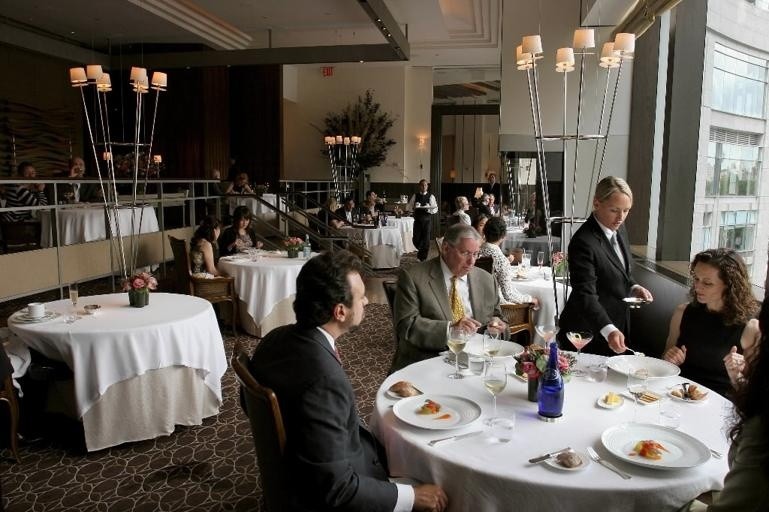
[668,384,708,402]
[393,394,482,430]
[543,450,592,471]
[597,393,624,410]
[622,390,662,405]
[624,298,645,308]
[605,355,681,380]
[462,338,524,360]
[386,385,423,399]
[601,423,711,470]
[224,256,241,261]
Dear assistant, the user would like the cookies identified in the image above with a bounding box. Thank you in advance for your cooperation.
[640,391,659,402]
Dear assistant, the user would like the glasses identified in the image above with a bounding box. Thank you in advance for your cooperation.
[446,244,483,260]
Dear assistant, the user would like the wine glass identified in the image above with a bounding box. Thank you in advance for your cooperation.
[627,368,648,418]
[537,251,544,271]
[448,327,466,379]
[567,332,593,376]
[535,325,560,349]
[484,330,501,380]
[484,359,507,426]
[69,281,82,320]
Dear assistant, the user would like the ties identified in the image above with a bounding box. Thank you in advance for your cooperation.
[489,184,494,193]
[332,344,343,365]
[610,233,626,272]
[450,277,465,325]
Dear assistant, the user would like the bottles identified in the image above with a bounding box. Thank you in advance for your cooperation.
[303,234,310,259]
[538,343,564,418]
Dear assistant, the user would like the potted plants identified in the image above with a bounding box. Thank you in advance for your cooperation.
[128,288,149,307]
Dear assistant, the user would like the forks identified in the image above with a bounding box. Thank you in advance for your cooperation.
[587,447,631,479]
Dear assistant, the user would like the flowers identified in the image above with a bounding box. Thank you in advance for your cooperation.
[282,236,304,247]
[121,273,158,291]
[514,347,577,379]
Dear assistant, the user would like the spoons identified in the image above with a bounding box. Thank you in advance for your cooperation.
[626,347,645,358]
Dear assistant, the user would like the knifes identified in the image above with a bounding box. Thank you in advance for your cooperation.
[528,446,571,464]
[480,318,497,327]
[428,431,483,446]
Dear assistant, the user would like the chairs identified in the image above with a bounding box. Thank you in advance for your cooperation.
[229,356,294,512]
[168,236,240,336]
[385,281,400,319]
[495,290,535,348]
[0,388,23,466]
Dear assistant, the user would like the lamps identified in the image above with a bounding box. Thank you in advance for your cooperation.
[325,134,363,203]
[515,28,635,330]
[70,63,168,281]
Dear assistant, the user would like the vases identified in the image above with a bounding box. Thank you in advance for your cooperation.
[287,247,299,258]
[526,380,550,402]
[288,249,299,258]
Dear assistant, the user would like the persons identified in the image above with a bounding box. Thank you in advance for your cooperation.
[403,179,438,261]
[389,224,509,375]
[480,193,490,206]
[475,217,539,311]
[335,197,361,224]
[555,176,653,356]
[470,184,490,215]
[473,214,490,241]
[317,197,345,235]
[662,248,761,393]
[49,156,103,202]
[359,191,386,216]
[1,162,47,242]
[217,206,264,255]
[239,252,449,512]
[487,192,500,217]
[449,196,473,224]
[680,255,768,512]
[481,170,503,205]
[224,173,254,194]
[190,215,238,326]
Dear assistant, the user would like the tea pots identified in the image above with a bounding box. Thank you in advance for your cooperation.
[416,202,421,207]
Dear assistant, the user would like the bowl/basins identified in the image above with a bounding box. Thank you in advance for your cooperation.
[84,304,101,314]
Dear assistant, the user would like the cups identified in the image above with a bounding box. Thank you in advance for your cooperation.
[522,254,531,268]
[249,247,257,262]
[544,268,551,281]
[504,216,525,231]
[28,303,44,318]
[426,203,429,206]
[590,357,608,382]
[494,409,515,443]
[469,350,484,376]
[659,396,681,428]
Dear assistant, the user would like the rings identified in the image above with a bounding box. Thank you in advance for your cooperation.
[737,359,743,364]
